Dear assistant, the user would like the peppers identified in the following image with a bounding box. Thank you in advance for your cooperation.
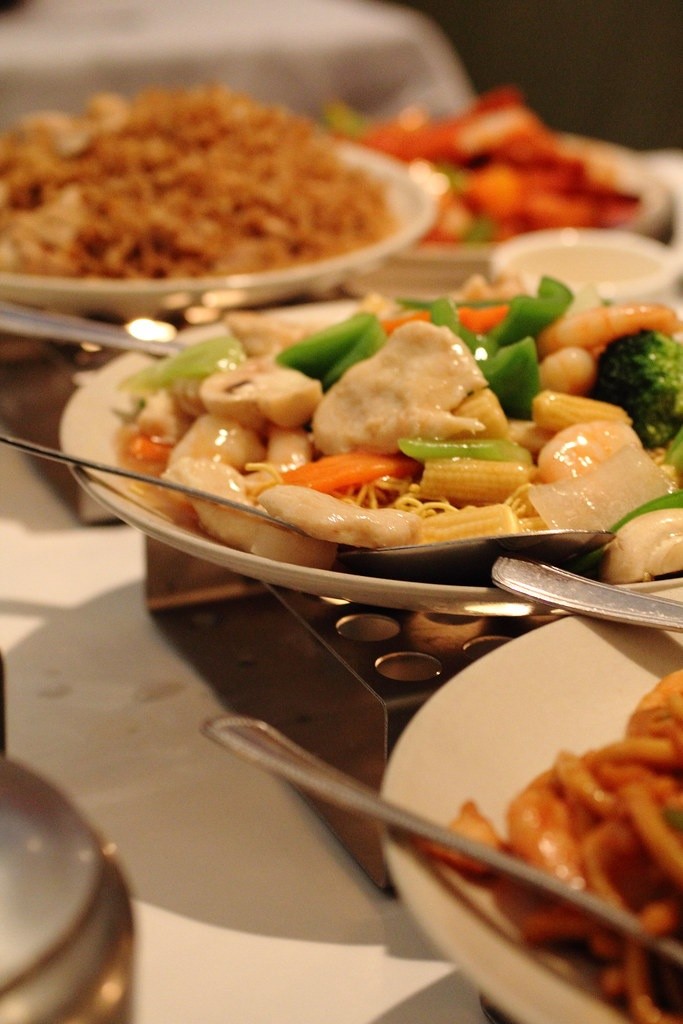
[274,273,576,427]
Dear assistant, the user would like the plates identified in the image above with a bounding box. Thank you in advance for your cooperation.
[1,145,439,325]
[344,131,676,295]
[376,583,683,1023]
[61,297,683,617]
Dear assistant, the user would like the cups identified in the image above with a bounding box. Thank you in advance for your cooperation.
[469,224,681,319]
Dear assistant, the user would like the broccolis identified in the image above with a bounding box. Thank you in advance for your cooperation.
[589,323,682,449]
